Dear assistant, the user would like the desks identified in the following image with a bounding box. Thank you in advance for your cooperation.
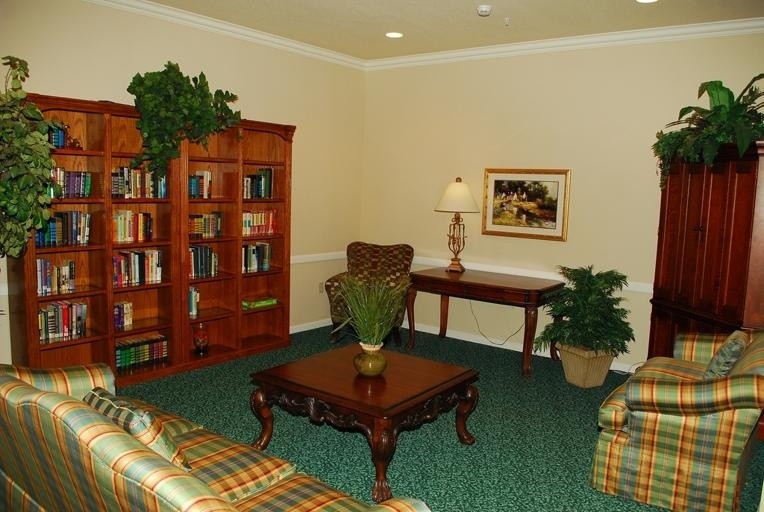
[404,266,566,378]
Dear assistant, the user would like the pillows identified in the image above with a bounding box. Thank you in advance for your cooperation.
[702,329,751,380]
[81,386,193,473]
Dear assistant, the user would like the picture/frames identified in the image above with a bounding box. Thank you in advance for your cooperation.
[481,167,572,242]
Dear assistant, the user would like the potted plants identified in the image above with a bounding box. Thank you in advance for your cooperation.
[530,264,636,390]
[327,269,415,379]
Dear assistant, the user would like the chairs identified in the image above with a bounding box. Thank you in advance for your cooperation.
[324,240,415,347]
[586,328,764,512]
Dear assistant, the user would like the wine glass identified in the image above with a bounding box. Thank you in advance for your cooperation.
[192,322,209,357]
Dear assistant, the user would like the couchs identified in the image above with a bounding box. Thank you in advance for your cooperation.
[0,360,434,512]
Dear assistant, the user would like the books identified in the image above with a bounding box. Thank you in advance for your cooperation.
[242,168,279,309]
[112,167,167,375]
[189,171,224,319]
[35,121,91,344]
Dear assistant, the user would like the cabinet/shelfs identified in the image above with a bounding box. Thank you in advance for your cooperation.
[648,139,764,444]
[7,92,116,389]
[105,97,183,388]
[241,115,295,358]
[181,108,243,369]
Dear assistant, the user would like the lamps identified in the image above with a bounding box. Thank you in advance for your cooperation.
[433,176,481,273]
